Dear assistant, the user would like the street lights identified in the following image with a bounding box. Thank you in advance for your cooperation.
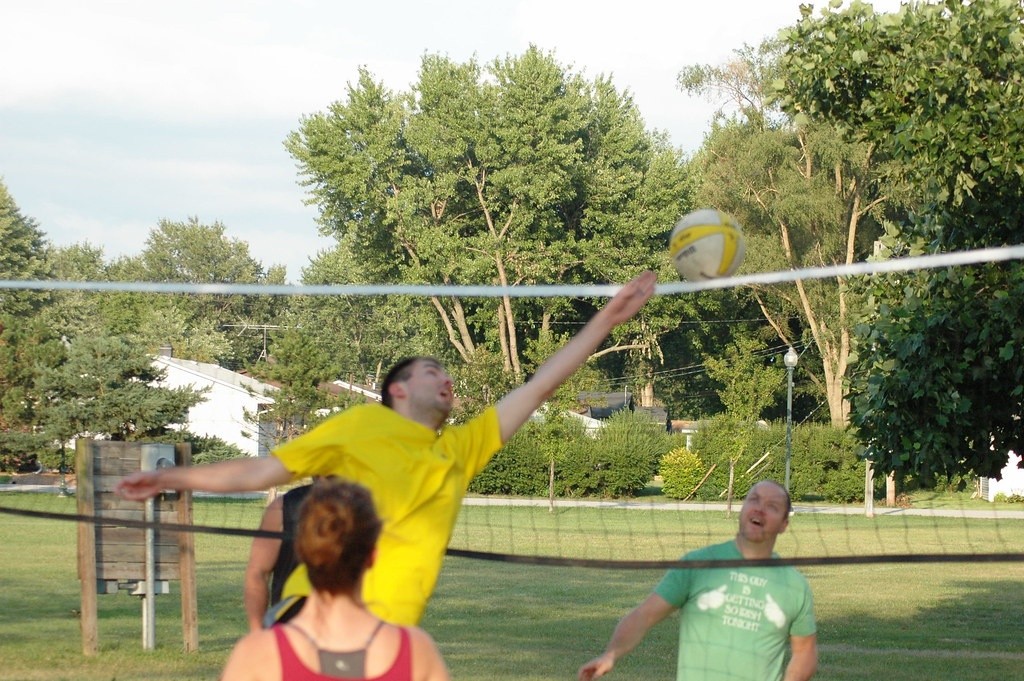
[783,347,799,492]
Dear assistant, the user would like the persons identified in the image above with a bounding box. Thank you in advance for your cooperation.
[579,478,818,681]
[243,471,340,630]
[113,268,657,627]
[218,481,454,681]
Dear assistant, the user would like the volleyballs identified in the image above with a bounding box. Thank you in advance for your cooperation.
[670,209,745,283]
